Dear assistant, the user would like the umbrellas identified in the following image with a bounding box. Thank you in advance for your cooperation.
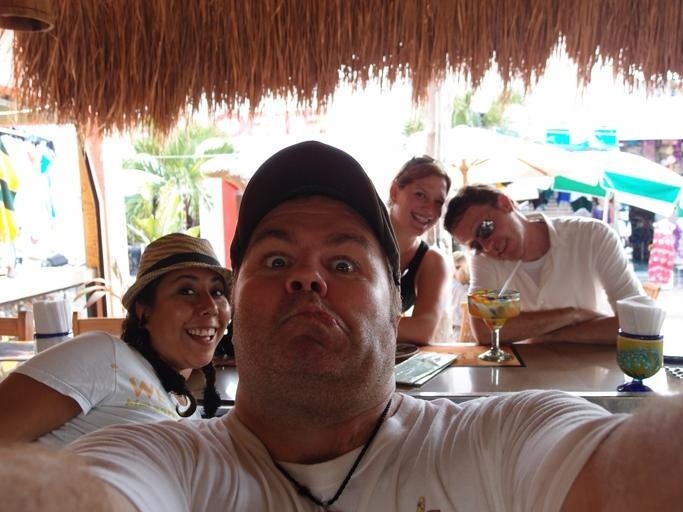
[379,131,567,201]
[548,142,683,225]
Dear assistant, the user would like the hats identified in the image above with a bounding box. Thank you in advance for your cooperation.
[230,141,401,285]
[122,233,234,312]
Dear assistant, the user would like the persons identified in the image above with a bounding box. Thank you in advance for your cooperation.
[443,182,647,345]
[385,154,452,345]
[537,191,555,211]
[0,139,683,512]
[590,197,610,223]
[1,232,241,448]
[451,250,468,340]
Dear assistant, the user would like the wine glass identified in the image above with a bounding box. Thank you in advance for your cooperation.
[614,328,662,392]
[467,289,522,364]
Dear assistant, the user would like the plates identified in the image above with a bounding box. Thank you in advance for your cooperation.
[394,343,419,362]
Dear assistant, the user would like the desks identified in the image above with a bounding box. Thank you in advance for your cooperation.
[176,337,683,417]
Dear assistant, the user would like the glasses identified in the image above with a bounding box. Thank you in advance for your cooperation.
[393,154,435,182]
[468,205,496,256]
